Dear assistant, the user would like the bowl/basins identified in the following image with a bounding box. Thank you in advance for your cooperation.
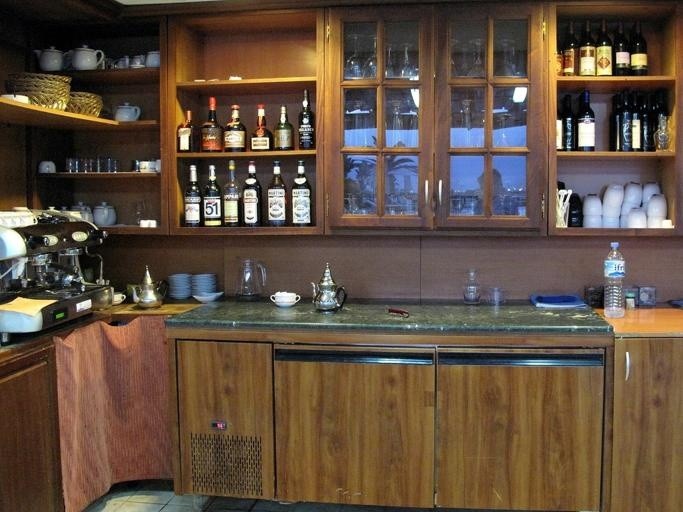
[138,161,155,174]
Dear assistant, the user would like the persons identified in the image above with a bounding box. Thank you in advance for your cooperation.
[474,170,506,216]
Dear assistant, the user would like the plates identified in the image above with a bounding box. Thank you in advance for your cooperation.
[167,272,225,303]
[129,64,144,69]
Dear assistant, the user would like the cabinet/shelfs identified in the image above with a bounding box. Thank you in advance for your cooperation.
[275,329,606,512]
[164,327,274,501]
[607,337,682,512]
[166,5,327,234]
[324,1,547,232]
[0,0,165,234]
[0,337,68,511]
[548,2,680,236]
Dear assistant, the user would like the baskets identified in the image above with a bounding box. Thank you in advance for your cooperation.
[10,72,102,117]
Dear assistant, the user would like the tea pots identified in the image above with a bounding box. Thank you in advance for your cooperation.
[234,257,267,303]
[111,101,140,123]
[130,265,168,310]
[71,200,117,226]
[32,43,105,72]
[307,261,347,313]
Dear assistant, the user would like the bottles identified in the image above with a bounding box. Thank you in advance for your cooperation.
[249,104,273,150]
[457,99,473,148]
[601,241,626,318]
[486,286,504,306]
[223,104,247,151]
[291,160,311,226]
[556,88,651,152]
[183,159,263,227]
[388,98,405,146]
[175,109,200,152]
[298,88,316,151]
[272,103,294,149]
[653,114,673,152]
[652,90,669,152]
[556,18,647,75]
[200,96,223,152]
[266,160,287,226]
[344,100,370,150]
[460,268,482,305]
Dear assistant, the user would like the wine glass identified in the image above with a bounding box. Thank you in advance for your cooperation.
[493,101,512,147]
[345,31,516,79]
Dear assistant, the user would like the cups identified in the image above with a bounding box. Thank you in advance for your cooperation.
[155,160,160,173]
[557,179,672,229]
[38,160,55,174]
[64,155,120,173]
[116,54,130,68]
[111,291,126,307]
[145,50,160,68]
[339,190,419,216]
[268,291,300,308]
[449,195,526,215]
[130,55,143,65]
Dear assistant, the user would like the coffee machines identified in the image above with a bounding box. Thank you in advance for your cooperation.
[0,210,113,344]
